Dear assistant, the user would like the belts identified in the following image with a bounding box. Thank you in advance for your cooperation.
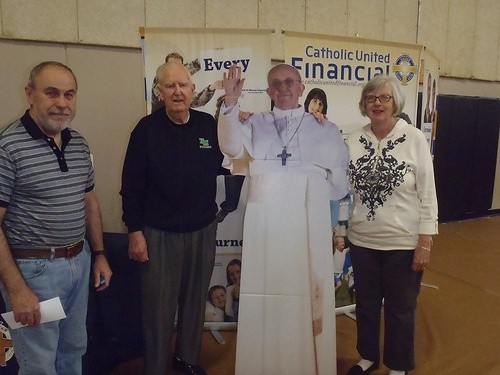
[10,240,84,259]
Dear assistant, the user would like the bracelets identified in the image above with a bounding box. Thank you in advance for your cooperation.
[93,249,108,257]
[416,243,432,253]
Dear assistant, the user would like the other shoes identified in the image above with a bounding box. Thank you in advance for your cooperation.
[347,356,378,375]
[388,369,409,375]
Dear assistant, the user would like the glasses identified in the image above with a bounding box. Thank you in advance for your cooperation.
[365,94,394,103]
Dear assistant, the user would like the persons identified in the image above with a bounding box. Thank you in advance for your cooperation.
[0,60,113,375]
[204,258,242,323]
[151,51,225,114]
[118,61,440,375]
[303,87,328,116]
[332,224,356,308]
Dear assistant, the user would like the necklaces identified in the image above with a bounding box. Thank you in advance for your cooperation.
[268,108,306,167]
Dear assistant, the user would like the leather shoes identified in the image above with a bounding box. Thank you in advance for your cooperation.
[172,355,208,375]
